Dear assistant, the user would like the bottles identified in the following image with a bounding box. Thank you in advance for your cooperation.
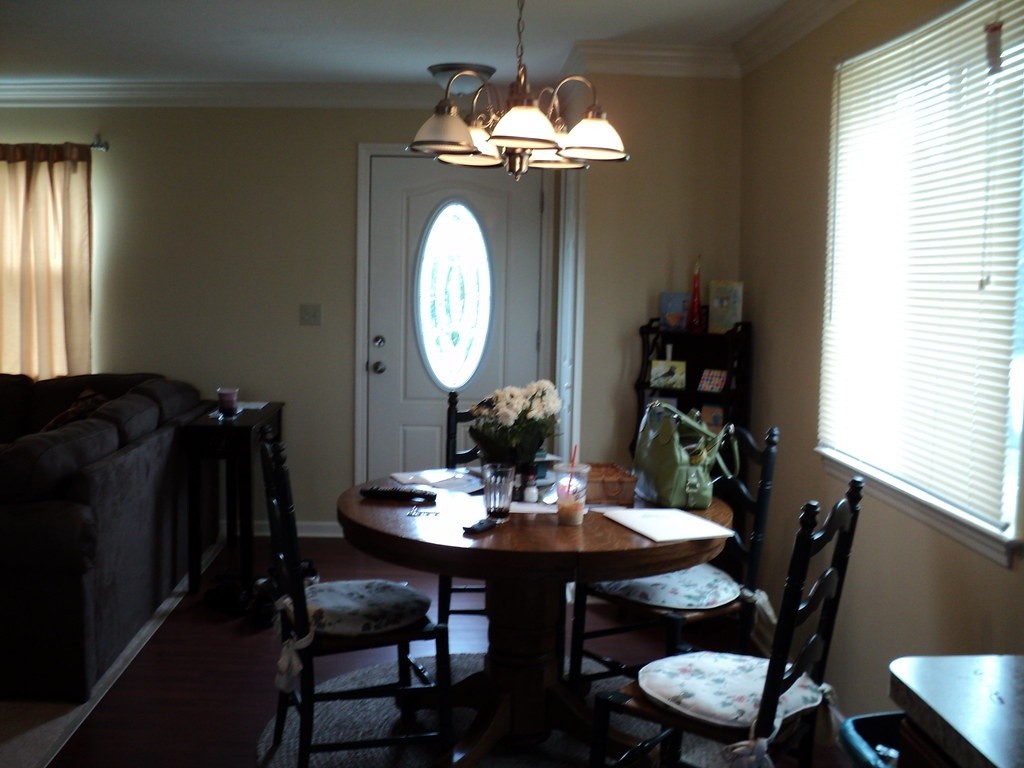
[523,480,538,502]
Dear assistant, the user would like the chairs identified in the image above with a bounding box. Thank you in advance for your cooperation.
[263,421,452,768]
[600,474,863,768]
[568,412,780,692]
[438,390,564,680]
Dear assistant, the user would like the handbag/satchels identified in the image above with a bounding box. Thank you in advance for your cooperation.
[630,402,741,509]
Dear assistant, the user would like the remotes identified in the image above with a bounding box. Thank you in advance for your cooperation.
[360,485,436,502]
[462,520,497,532]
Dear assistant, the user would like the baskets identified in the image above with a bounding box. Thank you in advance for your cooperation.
[585,461,637,506]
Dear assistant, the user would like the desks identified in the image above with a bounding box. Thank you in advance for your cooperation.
[184,399,285,621]
[335,466,733,768]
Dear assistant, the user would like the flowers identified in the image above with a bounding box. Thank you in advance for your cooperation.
[469,380,562,442]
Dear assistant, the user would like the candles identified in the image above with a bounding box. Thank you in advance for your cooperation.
[692,253,703,322]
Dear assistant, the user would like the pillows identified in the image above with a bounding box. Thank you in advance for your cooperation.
[41,395,107,434]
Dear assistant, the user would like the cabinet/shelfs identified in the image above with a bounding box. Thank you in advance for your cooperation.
[632,314,753,453]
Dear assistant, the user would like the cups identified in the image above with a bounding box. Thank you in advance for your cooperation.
[482,463,516,524]
[217,387,239,415]
[553,463,591,526]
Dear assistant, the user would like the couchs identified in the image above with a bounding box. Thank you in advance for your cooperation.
[0,372,202,701]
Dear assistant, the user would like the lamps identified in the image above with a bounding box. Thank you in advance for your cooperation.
[407,1,629,178]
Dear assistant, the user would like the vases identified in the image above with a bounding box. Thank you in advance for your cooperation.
[479,447,538,504]
